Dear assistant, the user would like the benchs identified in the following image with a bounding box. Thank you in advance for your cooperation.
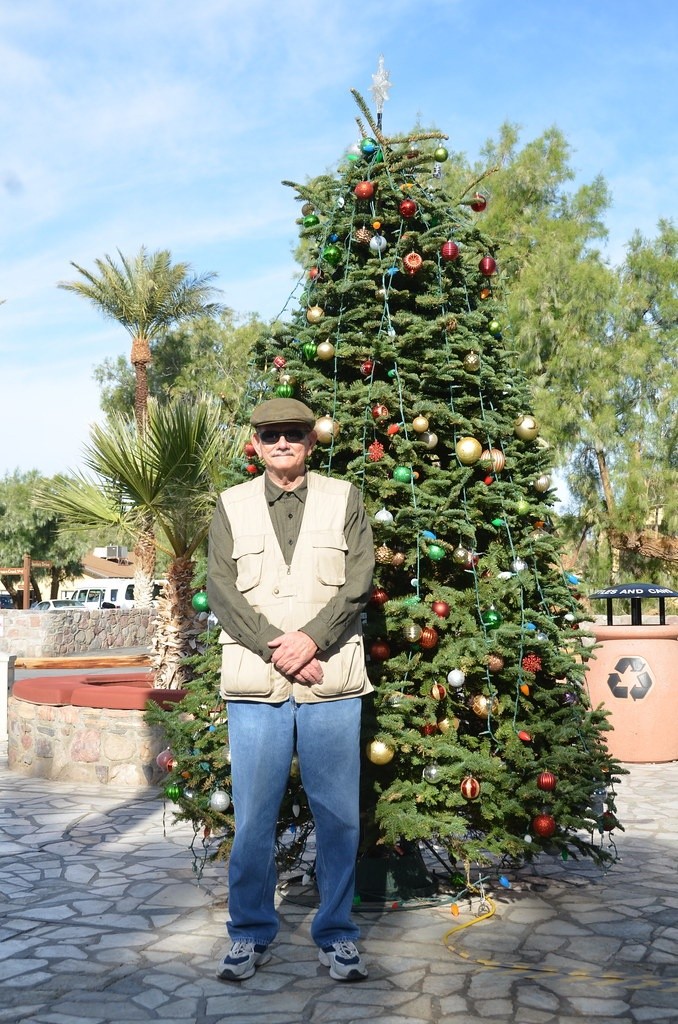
[8,672,226,789]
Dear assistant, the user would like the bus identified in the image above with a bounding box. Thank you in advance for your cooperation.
[67,578,171,610]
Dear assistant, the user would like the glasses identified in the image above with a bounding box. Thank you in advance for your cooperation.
[258,429,310,443]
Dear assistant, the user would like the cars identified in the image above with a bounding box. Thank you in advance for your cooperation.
[0,594,15,609]
[30,600,87,611]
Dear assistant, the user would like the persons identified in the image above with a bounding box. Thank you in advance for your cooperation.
[206,398,374,981]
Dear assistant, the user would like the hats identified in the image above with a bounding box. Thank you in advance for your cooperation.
[249,397,316,430]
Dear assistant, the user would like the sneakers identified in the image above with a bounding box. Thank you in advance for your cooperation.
[318,940,369,981]
[216,941,272,981]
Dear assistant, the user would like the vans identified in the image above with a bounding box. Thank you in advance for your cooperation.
[102,581,166,609]
